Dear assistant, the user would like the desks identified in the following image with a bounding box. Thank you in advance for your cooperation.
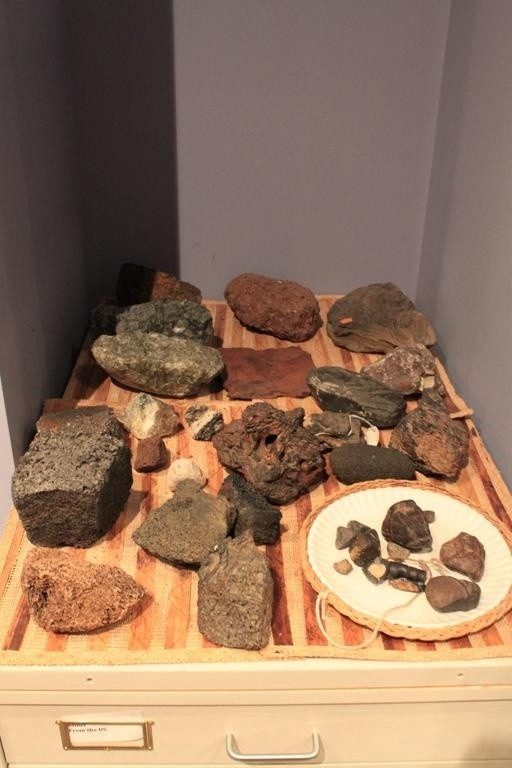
[0,298,511,768]
